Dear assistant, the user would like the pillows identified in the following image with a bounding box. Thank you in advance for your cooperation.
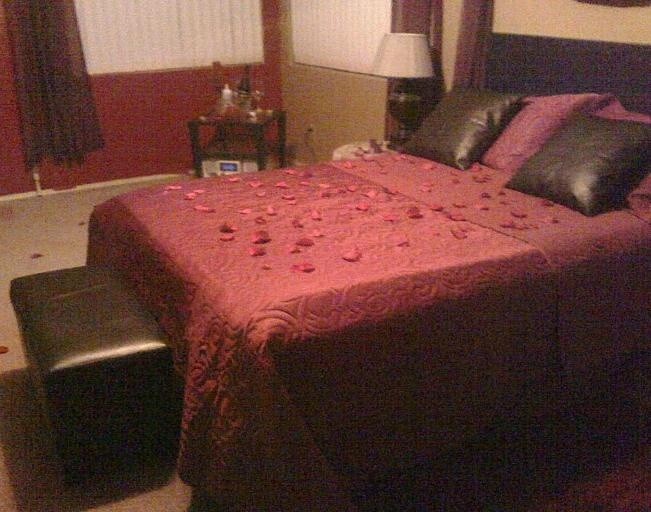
[404,90,651,219]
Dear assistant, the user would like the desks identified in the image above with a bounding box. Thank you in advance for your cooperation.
[188,105,287,177]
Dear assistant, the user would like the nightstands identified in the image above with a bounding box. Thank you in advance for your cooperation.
[334,140,404,159]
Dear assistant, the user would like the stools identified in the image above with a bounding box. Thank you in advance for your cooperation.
[9,263,177,486]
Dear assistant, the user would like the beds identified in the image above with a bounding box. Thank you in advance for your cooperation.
[84,32,651,512]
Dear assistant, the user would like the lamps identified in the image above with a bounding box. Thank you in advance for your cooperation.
[370,32,438,146]
